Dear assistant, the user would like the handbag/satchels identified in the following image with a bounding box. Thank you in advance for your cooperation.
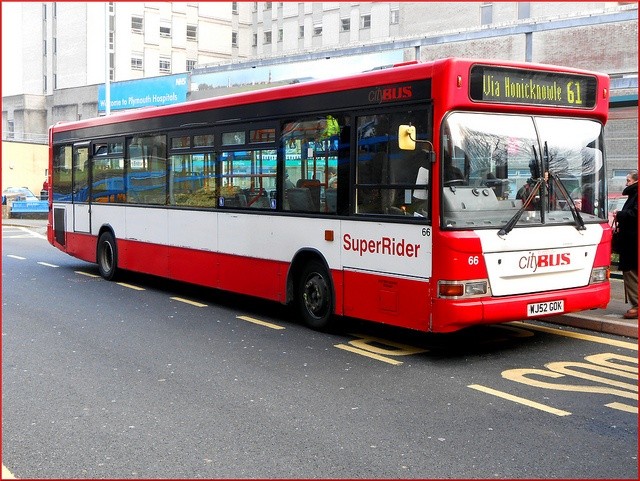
[611,219,620,255]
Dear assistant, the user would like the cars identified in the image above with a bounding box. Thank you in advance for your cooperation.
[576,193,626,239]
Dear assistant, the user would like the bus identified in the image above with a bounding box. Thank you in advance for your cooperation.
[47,58,611,334]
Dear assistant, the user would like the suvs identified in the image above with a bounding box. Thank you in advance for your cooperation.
[4,186,38,202]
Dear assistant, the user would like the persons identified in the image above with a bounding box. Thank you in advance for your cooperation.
[282,174,296,191]
[401,133,450,215]
[516,160,560,211]
[324,167,337,189]
[612,171,639,320]
[572,201,582,212]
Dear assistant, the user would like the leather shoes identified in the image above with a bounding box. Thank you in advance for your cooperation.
[624,308,637,319]
